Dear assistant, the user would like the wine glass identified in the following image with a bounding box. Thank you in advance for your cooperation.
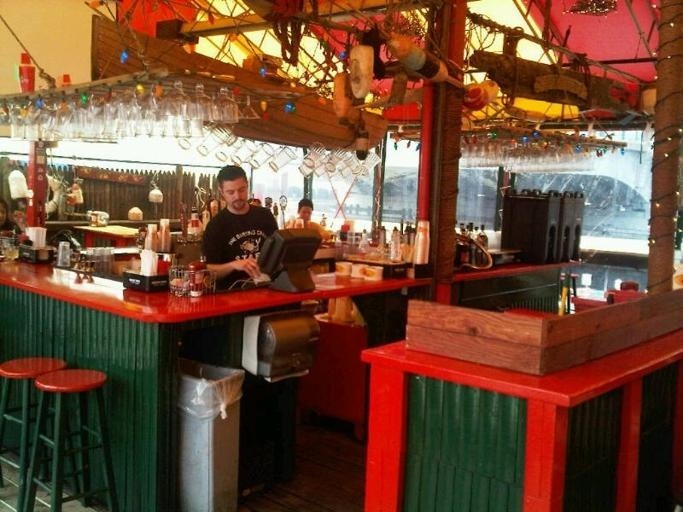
[0,86,261,141]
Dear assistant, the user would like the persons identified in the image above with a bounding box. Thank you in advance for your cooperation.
[200,164,280,289]
[284,197,330,239]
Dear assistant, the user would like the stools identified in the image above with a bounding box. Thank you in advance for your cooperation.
[561,272,579,312]
[24,368,120,512]
[1,358,79,511]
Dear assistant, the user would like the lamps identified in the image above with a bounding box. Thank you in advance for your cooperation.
[356,121,368,160]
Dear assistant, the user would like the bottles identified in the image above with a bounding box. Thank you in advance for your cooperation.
[454,222,489,269]
[144,224,158,253]
[188,261,206,297]
[18,52,36,94]
[157,217,172,253]
[318,216,430,264]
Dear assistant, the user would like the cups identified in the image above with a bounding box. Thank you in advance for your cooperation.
[56,241,71,268]
[334,262,352,275]
[171,121,382,180]
[350,264,369,278]
[364,266,384,281]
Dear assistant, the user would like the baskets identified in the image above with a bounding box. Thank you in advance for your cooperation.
[168,264,218,299]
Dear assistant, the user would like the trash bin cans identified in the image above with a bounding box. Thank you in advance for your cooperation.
[178,357,246,512]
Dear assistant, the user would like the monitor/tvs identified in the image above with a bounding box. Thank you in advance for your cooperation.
[257,228,322,274]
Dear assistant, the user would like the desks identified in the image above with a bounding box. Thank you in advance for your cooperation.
[74,224,139,247]
[314,243,339,273]
